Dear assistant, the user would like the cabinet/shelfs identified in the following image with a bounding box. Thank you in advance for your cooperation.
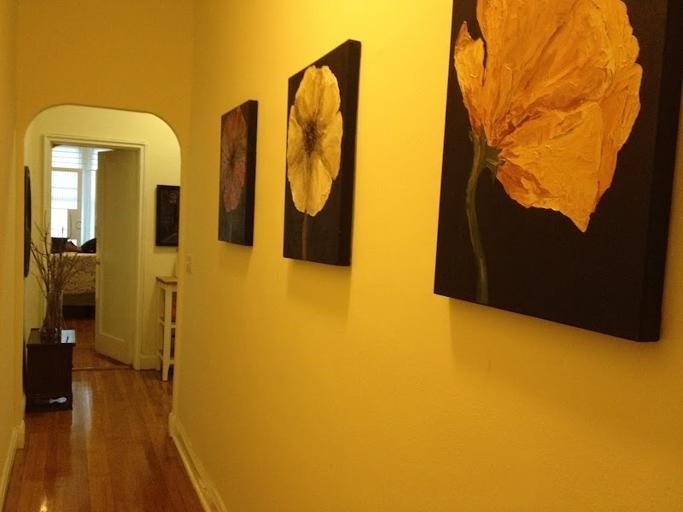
[26,327,76,412]
[155,275,177,381]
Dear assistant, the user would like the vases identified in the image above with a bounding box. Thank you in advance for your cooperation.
[38,289,64,338]
